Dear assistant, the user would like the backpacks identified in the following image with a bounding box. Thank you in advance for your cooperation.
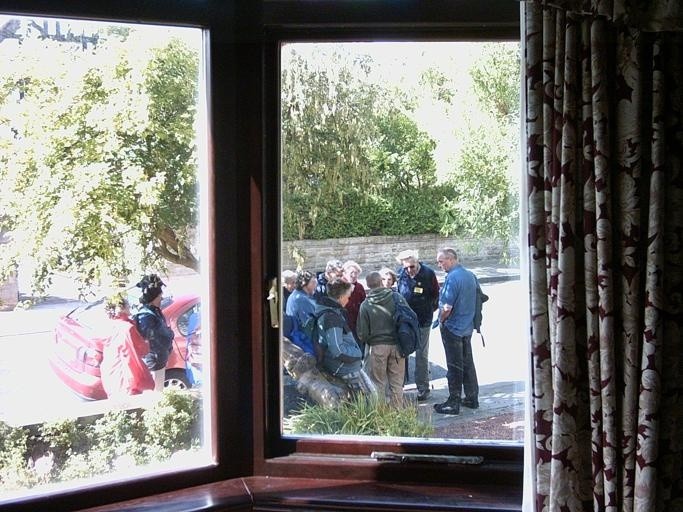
[130,304,157,320]
[303,309,345,366]
[472,272,489,333]
[391,292,422,359]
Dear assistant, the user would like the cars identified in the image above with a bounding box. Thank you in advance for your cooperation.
[39,274,204,403]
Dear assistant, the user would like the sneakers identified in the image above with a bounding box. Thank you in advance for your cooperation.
[460,396,480,410]
[433,402,460,415]
[416,389,433,401]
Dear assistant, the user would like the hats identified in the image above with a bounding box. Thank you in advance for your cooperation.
[139,284,162,304]
[135,275,167,289]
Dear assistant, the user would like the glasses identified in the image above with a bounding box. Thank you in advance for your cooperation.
[401,265,416,271]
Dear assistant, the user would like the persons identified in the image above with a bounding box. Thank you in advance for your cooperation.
[100,291,150,399]
[131,275,174,392]
[281,247,484,415]
